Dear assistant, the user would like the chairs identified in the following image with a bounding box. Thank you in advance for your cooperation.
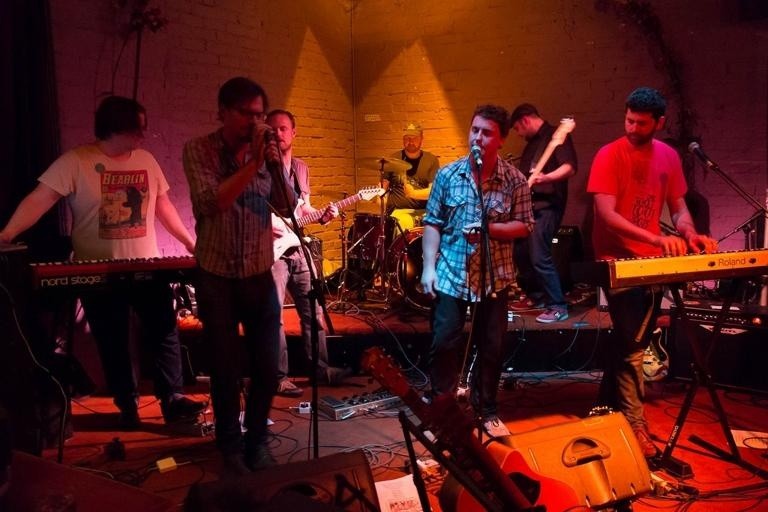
[480,415,510,437]
[635,428,657,458]
[509,297,545,311]
[317,367,352,386]
[159,397,209,421]
[276,380,303,396]
[536,307,569,323]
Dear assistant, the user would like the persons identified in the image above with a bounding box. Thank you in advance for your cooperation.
[507,106,578,324]
[583,88,719,461]
[181,77,297,474]
[265,107,343,397]
[376,122,440,219]
[419,103,537,439]
[0,93,204,431]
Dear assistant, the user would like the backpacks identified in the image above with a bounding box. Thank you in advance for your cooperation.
[386,226,436,313]
[350,212,397,260]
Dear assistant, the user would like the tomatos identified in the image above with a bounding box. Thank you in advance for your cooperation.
[594,248,767,288]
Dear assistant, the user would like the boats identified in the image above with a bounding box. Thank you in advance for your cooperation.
[304,234,322,281]
[352,213,397,260]
[551,226,584,292]
[441,413,652,512]
[181,448,380,511]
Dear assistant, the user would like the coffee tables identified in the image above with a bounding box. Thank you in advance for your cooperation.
[356,157,413,171]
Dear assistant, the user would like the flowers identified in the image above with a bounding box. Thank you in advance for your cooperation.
[471,144,483,166]
[688,141,718,170]
[264,127,274,141]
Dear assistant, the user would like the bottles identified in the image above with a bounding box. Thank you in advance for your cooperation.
[402,122,423,137]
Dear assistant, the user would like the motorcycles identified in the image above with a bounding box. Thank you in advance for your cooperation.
[324,214,368,315]
[359,172,390,299]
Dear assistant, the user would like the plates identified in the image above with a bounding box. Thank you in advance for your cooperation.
[658,278,747,468]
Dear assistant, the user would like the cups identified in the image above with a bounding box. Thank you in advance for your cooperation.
[224,104,269,122]
